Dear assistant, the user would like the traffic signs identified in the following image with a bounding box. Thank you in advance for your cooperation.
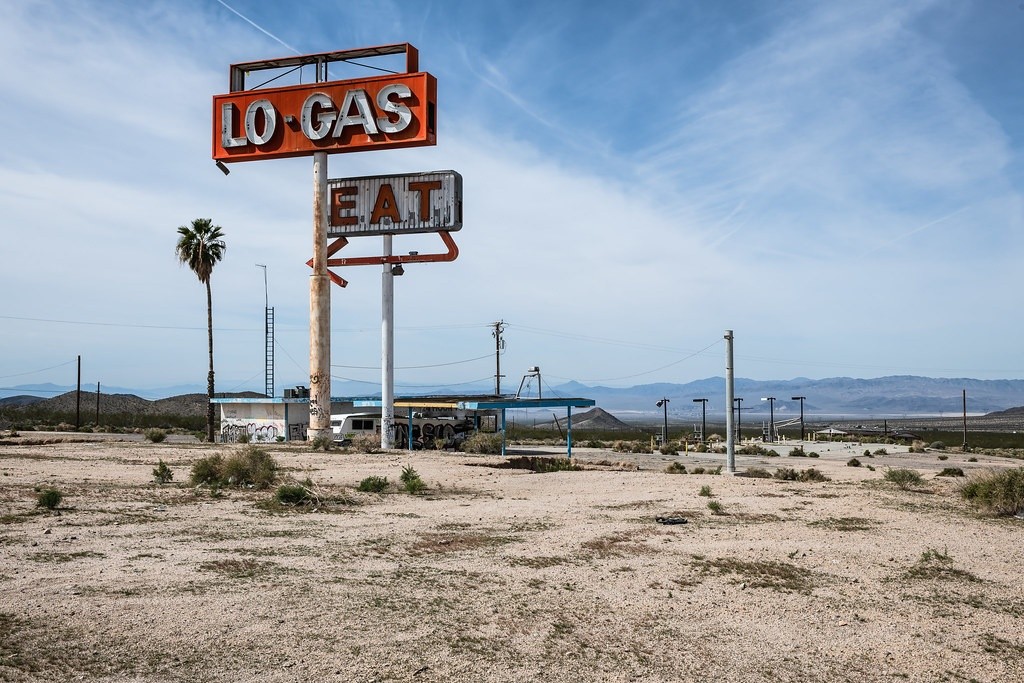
[306,170,462,288]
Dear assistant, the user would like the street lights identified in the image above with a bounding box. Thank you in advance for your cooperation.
[656,397,670,444]
[792,396,807,441]
[761,397,776,443]
[255,264,267,310]
[693,399,708,443]
[734,398,744,444]
